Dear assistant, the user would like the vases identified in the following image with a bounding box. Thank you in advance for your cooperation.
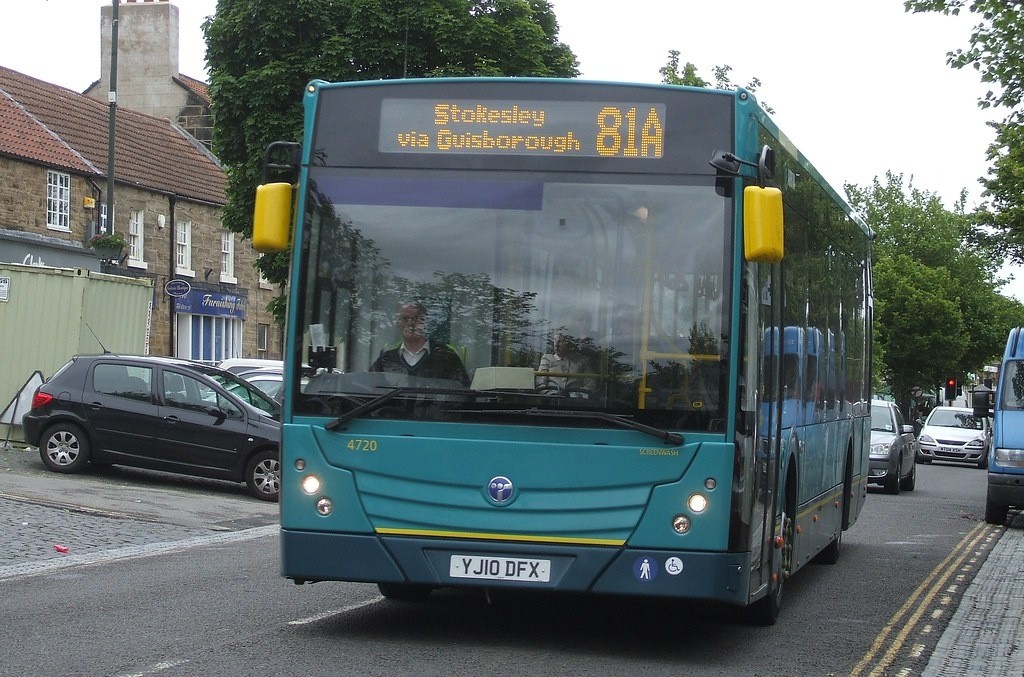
[94,244,123,259]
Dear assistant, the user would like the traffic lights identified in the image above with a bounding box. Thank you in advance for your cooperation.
[946,377,956,401]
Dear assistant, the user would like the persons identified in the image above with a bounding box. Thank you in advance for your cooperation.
[616,305,637,335]
[568,307,599,357]
[536,324,597,399]
[368,302,471,387]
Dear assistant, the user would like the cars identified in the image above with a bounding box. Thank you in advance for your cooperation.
[203,358,347,421]
[867,398,917,492]
[916,406,992,469]
[22,351,282,502]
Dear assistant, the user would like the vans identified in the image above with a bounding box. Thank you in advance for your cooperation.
[973,326,1024,524]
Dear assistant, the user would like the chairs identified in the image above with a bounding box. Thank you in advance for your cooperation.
[169,374,189,409]
[382,342,466,367]
[164,376,174,407]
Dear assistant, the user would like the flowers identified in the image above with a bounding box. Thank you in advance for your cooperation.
[90,233,127,246]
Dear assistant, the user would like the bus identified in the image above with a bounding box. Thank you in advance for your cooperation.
[251,77,876,627]
